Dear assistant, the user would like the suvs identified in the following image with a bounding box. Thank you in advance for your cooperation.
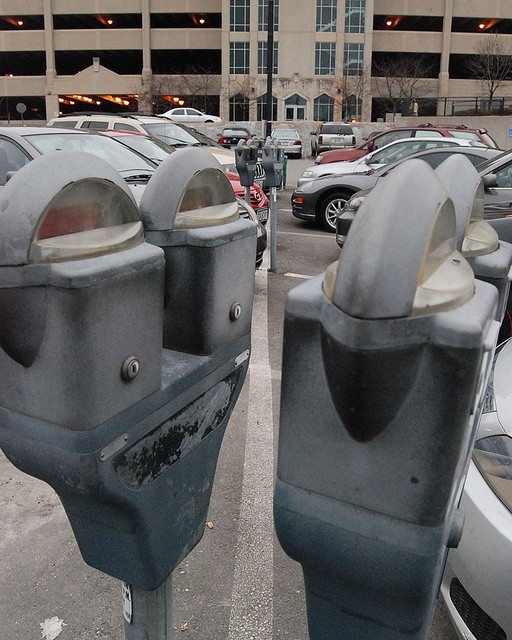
[48,115,266,192]
[310,122,357,156]
[315,125,501,163]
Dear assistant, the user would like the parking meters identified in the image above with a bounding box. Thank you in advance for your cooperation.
[275,156,512,640]
[2,147,259,639]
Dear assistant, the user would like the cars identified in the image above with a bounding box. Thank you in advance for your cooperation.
[157,108,225,124]
[96,129,270,226]
[297,138,488,188]
[269,128,301,159]
[337,150,512,254]
[217,127,256,145]
[443,340,510,632]
[291,147,508,230]
[0,127,267,270]
